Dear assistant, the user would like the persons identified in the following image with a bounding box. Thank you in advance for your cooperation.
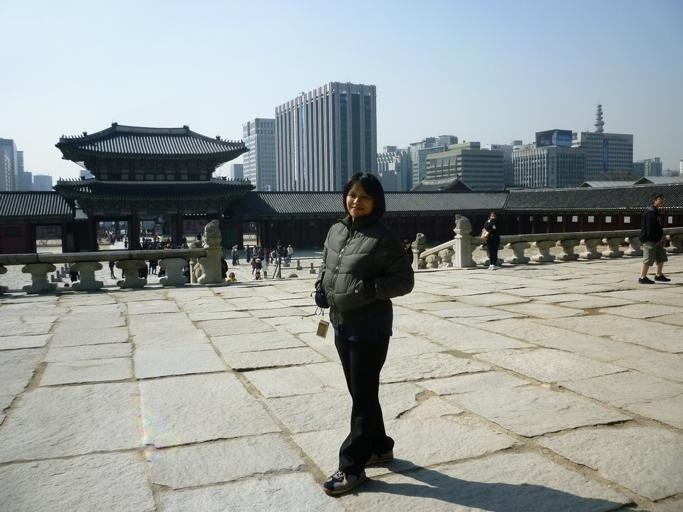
[100,226,300,281]
[481,210,501,271]
[312,174,414,495]
[638,193,672,284]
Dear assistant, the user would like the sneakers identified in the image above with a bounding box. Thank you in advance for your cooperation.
[639,277,655,284]
[323,468,367,494]
[655,275,671,281]
[363,448,393,466]
